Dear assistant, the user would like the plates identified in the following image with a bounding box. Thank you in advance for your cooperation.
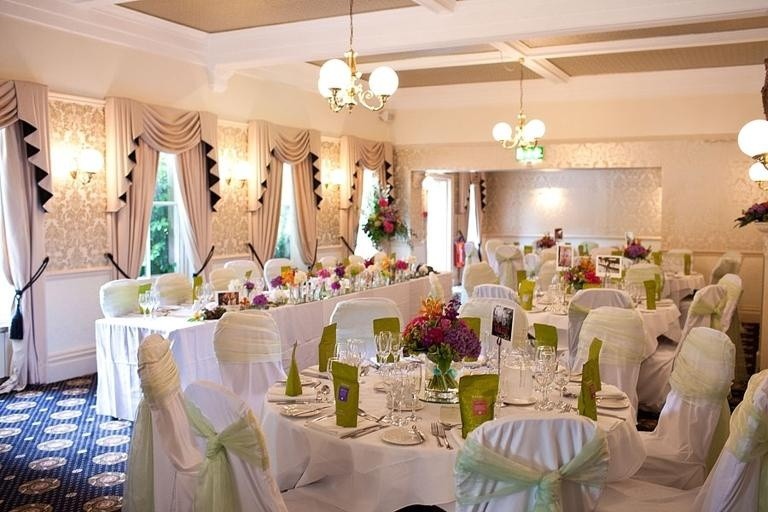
[280,409,321,418]
[596,398,629,408]
[382,428,424,445]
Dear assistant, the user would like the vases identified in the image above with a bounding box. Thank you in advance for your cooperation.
[753,222,768,232]
[244,289,252,299]
[255,304,264,310]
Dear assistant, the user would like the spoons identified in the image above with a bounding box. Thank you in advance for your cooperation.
[321,385,331,400]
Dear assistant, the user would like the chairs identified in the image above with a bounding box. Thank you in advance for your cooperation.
[348,254,362,264]
[638,284,729,413]
[473,284,520,305]
[592,248,622,258]
[461,298,529,354]
[486,239,504,271]
[629,326,736,489]
[539,259,556,291]
[209,268,235,291]
[560,288,635,371]
[463,241,480,265]
[225,260,262,292]
[457,413,611,512]
[591,247,617,259]
[138,334,207,509]
[667,248,695,273]
[264,257,292,289]
[571,305,646,426]
[461,262,499,297]
[182,379,343,511]
[625,262,664,295]
[155,272,193,303]
[580,240,599,250]
[317,256,337,269]
[494,245,524,290]
[711,251,745,285]
[99,279,140,317]
[717,272,743,334]
[211,309,288,418]
[596,369,768,512]
[428,271,444,301]
[329,297,404,362]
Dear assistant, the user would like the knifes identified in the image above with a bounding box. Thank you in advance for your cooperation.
[352,426,389,439]
[573,407,627,421]
[340,425,381,440]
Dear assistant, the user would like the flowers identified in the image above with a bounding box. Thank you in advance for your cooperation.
[536,233,555,248]
[295,271,307,284]
[282,270,294,285]
[399,290,481,392]
[271,276,285,287]
[731,201,768,227]
[252,293,269,306]
[316,268,330,280]
[624,236,652,260]
[334,263,346,278]
[396,259,409,271]
[244,282,253,289]
[562,256,602,290]
[363,183,416,251]
[331,281,341,288]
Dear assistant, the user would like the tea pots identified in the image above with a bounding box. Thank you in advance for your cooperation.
[504,356,537,405]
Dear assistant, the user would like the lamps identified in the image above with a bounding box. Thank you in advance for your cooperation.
[737,119,768,193]
[491,58,547,150]
[316,1,399,115]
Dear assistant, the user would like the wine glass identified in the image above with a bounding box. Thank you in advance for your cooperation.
[374,331,392,365]
[195,284,214,302]
[327,337,366,398]
[535,345,572,413]
[140,290,159,320]
[381,364,422,427]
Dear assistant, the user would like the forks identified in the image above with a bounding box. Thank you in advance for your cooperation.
[438,425,450,447]
[431,422,443,447]
